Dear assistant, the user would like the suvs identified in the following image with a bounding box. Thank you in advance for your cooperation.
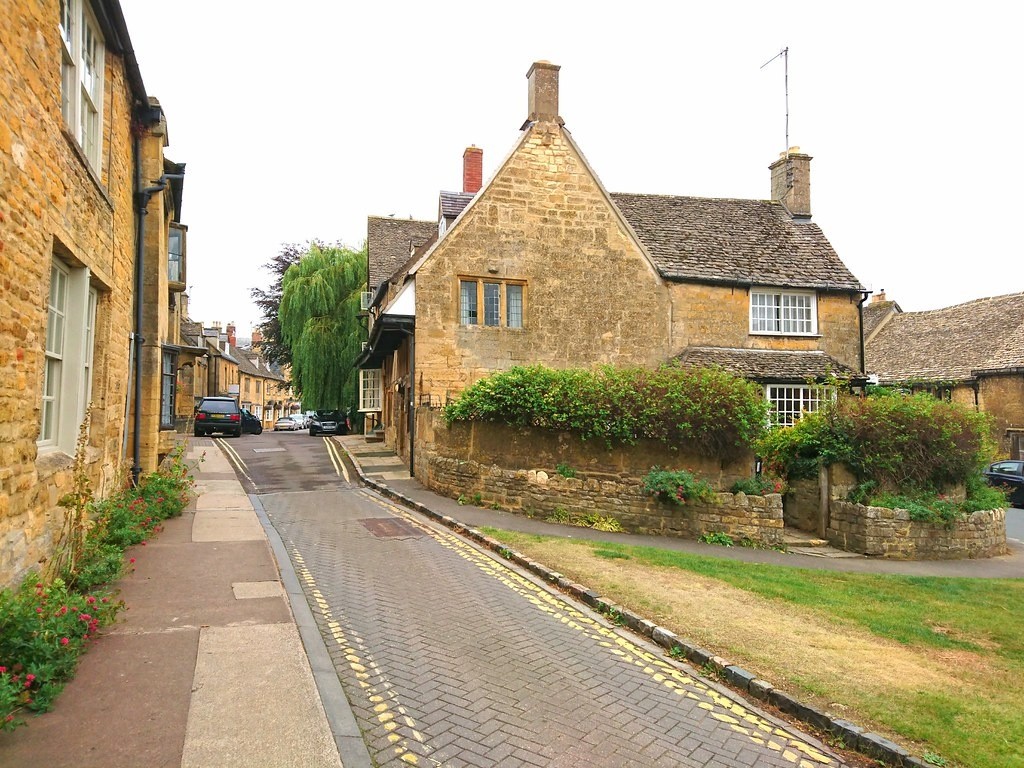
[194,396,245,437]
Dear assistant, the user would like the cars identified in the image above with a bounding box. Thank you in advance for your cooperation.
[274,416,300,431]
[309,409,352,436]
[979,459,1024,510]
[240,408,263,435]
[287,410,315,430]
